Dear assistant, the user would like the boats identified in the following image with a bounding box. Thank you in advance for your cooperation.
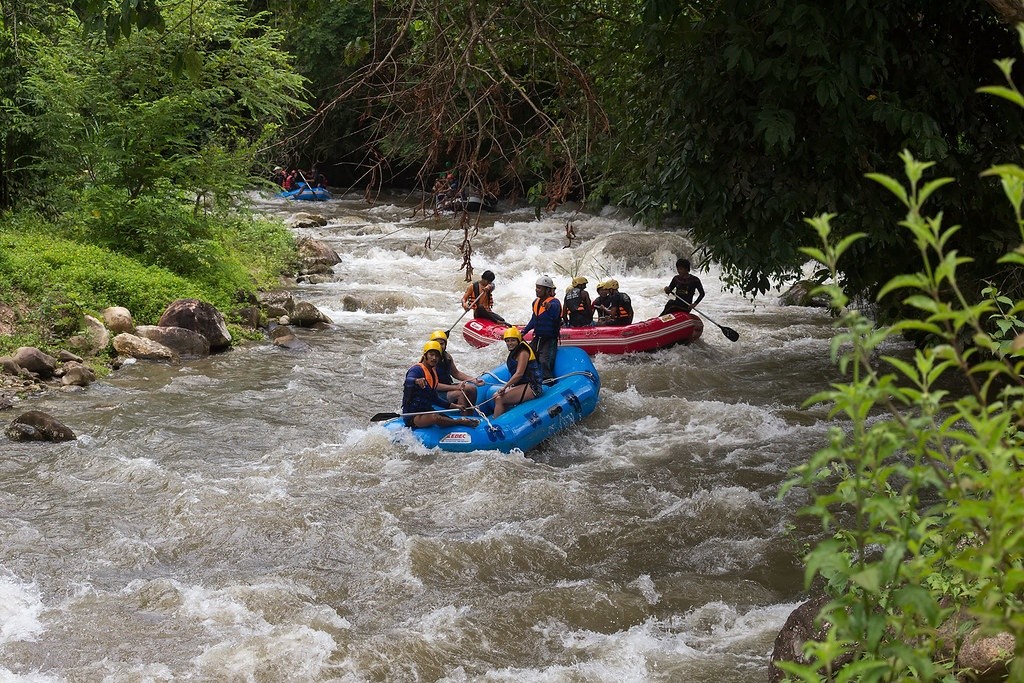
[461,312,703,356]
[274,181,333,201]
[437,195,494,213]
[379,345,601,457]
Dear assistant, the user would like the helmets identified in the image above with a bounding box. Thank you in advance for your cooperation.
[553,284,556,289]
[603,279,619,290]
[430,331,447,343]
[424,341,442,355]
[597,282,605,291]
[535,275,553,289]
[503,328,521,342]
[572,277,588,287]
[565,285,574,294]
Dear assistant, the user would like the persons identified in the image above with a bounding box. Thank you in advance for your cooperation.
[492,328,542,418]
[553,277,633,327]
[521,275,563,387]
[433,162,479,202]
[658,258,705,317]
[273,167,327,199]
[461,270,512,327]
[401,331,484,427]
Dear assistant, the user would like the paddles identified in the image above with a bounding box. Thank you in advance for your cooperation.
[299,170,319,200]
[669,290,740,343]
[369,408,463,421]
[444,290,485,338]
